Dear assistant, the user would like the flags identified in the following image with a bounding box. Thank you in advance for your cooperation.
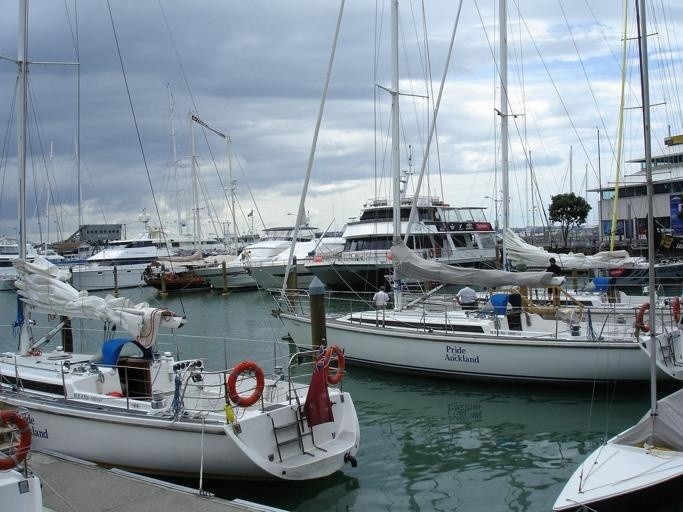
[248,210,253,217]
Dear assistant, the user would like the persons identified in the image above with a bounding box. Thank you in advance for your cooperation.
[456,285,479,310]
[544,258,562,300]
[372,286,390,309]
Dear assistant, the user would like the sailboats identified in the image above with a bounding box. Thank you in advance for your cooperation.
[552,0,683,512]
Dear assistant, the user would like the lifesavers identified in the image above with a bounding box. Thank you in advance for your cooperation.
[0,410,30,470]
[638,305,650,332]
[229,361,264,407]
[673,297,680,323]
[324,345,344,383]
